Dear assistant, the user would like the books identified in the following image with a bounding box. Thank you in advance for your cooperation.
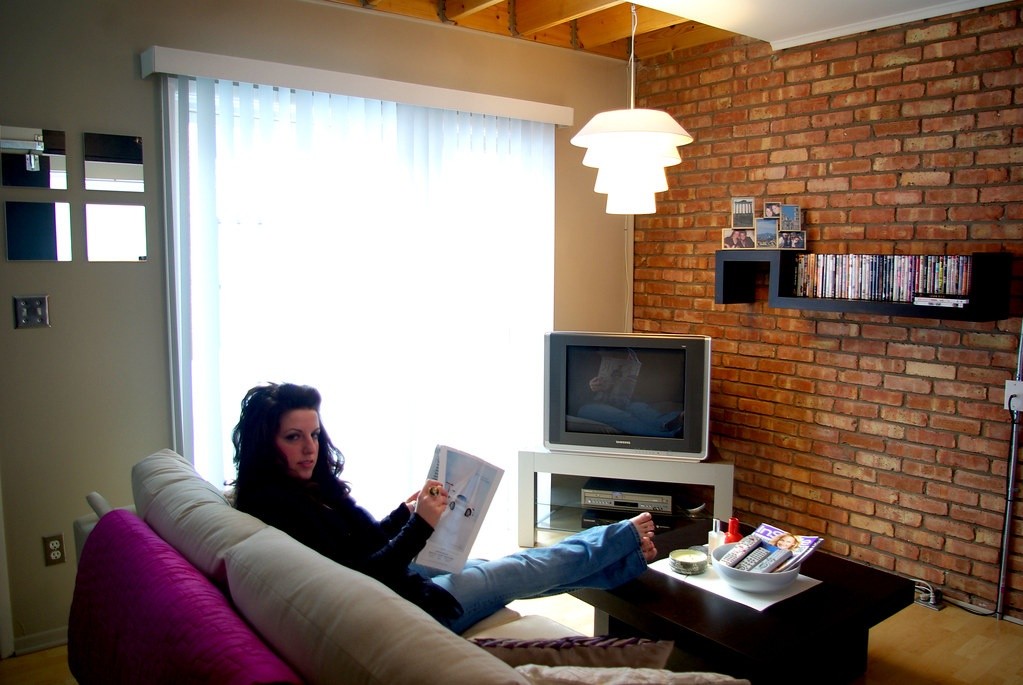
[754,523,824,573]
[594,349,641,407]
[791,252,972,307]
[411,444,505,575]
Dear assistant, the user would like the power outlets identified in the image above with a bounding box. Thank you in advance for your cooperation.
[915,595,947,612]
[42,534,65,566]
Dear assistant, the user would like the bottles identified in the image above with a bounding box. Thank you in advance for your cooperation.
[708,518,726,556]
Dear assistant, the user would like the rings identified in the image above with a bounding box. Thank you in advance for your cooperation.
[411,500,416,507]
[428,487,440,497]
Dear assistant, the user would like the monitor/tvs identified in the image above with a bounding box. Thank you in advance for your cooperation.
[543,331,711,463]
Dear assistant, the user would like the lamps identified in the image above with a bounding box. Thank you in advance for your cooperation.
[569,5,695,214]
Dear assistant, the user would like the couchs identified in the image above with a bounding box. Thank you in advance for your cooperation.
[67,447,752,685]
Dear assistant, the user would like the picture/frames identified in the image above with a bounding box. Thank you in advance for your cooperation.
[722,196,807,250]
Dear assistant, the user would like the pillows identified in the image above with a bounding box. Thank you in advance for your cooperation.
[469,636,752,685]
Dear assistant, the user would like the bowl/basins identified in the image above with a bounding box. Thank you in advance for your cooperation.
[711,542,801,594]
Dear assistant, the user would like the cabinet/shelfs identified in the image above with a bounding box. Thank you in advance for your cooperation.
[518,450,734,548]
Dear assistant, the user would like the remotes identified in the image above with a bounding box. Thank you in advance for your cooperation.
[733,546,770,571]
[718,535,762,566]
[751,549,793,572]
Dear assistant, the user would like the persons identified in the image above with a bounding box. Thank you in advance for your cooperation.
[773,533,800,552]
[766,203,780,216]
[566,348,684,437]
[232,382,657,637]
[778,232,804,248]
[724,230,754,248]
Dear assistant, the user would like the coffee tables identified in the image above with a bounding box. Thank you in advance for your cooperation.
[568,518,916,685]
